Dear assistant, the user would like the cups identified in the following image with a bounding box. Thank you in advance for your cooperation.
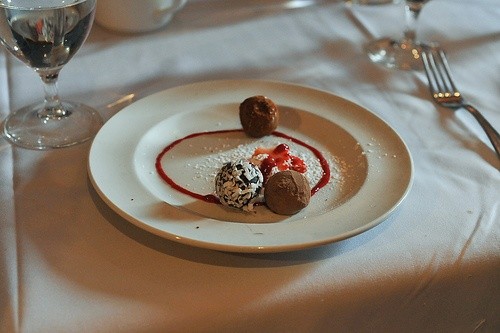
[94,0,188,34]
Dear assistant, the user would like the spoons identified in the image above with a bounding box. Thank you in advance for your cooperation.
[342,7,398,71]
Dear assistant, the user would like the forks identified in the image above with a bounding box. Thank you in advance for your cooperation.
[420,49,500,159]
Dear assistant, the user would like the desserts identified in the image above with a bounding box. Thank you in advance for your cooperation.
[216,160,263,212]
[239,96,279,138]
[264,169,311,215]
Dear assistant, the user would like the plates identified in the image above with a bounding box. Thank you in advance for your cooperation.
[85,80,415,252]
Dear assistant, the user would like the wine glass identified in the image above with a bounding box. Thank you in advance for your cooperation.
[392,0,441,67]
[0,0,105,150]
